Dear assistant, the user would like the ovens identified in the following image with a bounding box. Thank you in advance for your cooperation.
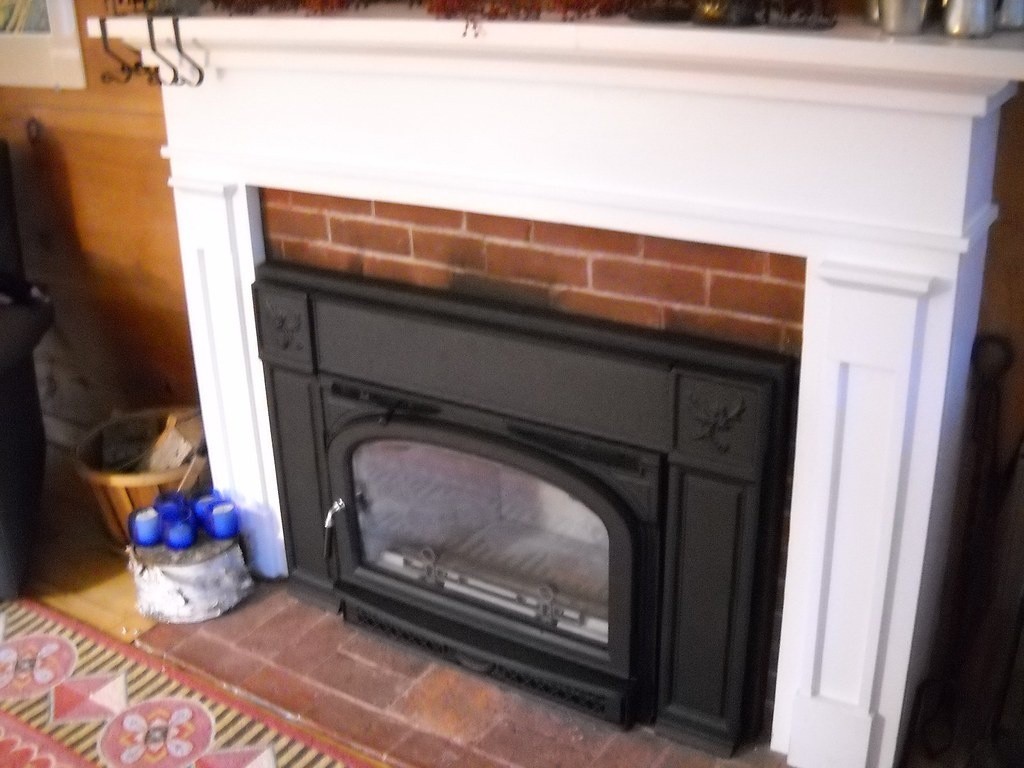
[250,262,800,758]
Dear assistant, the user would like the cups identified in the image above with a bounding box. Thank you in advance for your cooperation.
[128,488,239,550]
